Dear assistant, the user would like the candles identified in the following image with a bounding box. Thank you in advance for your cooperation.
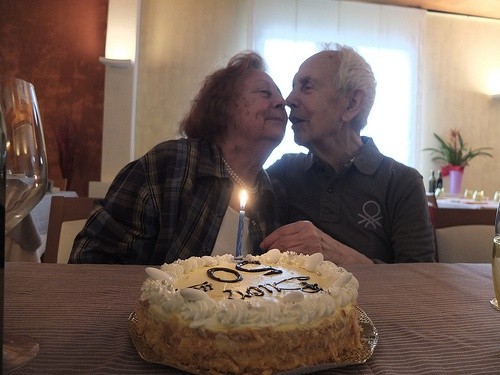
[236,189,249,258]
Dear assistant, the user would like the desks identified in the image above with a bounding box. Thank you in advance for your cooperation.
[427,197,499,264]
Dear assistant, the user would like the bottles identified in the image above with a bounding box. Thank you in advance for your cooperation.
[436,170,443,189]
[428,169,436,193]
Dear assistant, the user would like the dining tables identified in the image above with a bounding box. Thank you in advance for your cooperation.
[2,262,500,375]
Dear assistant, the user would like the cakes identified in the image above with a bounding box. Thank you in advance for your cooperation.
[133,248,363,375]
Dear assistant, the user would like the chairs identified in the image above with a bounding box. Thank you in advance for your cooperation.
[45,196,104,264]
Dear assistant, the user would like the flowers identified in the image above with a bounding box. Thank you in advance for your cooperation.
[422,128,493,166]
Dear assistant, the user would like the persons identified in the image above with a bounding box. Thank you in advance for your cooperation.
[260,45,436,264]
[67,52,288,265]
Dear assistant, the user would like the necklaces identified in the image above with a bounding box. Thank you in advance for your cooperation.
[222,157,260,194]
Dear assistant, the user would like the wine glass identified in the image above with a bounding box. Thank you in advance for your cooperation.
[1,75,49,373]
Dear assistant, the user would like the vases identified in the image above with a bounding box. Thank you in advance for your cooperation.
[448,166,464,195]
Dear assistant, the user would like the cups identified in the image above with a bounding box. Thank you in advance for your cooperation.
[490,201,500,313]
